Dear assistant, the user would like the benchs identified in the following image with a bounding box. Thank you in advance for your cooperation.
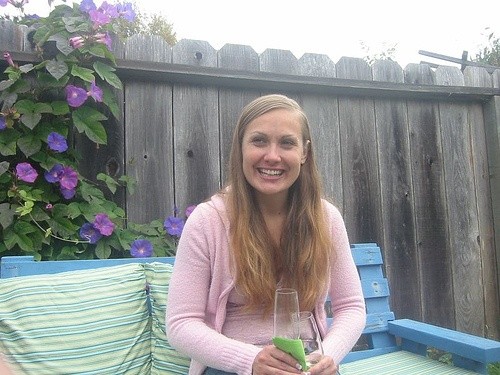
[0,243,499,374]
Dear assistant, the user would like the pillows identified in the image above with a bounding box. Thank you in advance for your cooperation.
[0,264,150,374]
[143,262,192,375]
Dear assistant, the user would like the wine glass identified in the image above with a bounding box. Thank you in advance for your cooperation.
[291,311,340,375]
[274,288,301,341]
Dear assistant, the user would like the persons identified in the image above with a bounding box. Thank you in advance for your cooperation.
[165,91,369,375]
